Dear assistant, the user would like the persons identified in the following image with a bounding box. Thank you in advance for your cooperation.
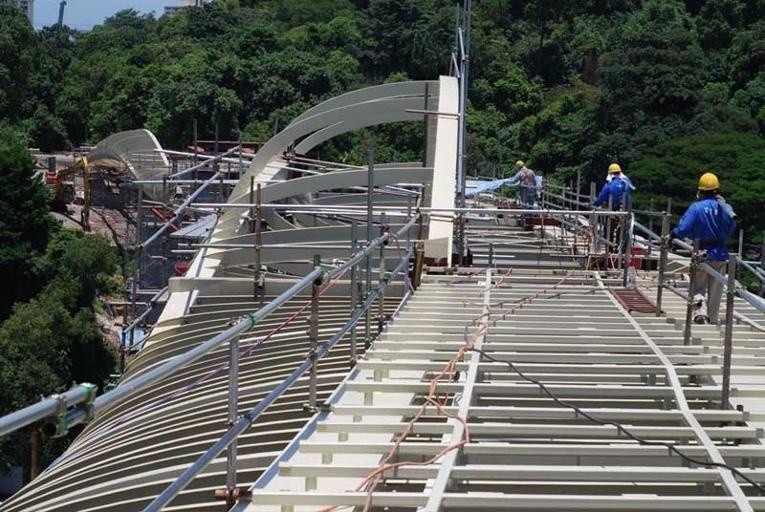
[502,160,538,231]
[589,163,637,267]
[665,172,739,325]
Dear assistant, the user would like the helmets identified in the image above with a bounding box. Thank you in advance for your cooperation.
[609,163,622,173]
[698,172,720,191]
[516,160,524,167]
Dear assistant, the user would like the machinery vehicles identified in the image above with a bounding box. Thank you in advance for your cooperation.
[39,154,94,234]
